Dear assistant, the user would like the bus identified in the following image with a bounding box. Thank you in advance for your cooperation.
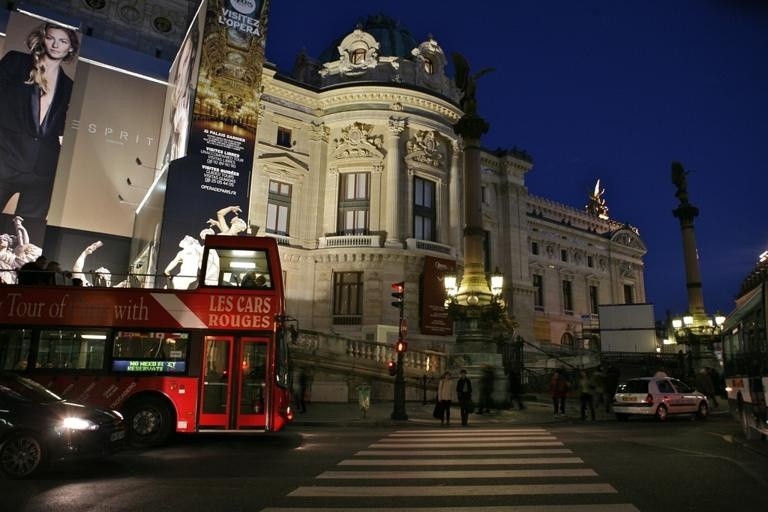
[0,234,299,449]
[721,280,768,442]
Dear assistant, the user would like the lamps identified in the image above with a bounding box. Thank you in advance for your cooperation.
[117,157,162,207]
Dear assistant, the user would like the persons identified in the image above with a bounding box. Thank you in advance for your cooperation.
[456,369,472,425]
[438,371,455,426]
[653,367,668,392]
[505,361,526,410]
[0,215,43,284]
[73,241,127,288]
[475,362,494,415]
[17,255,85,287]
[688,367,721,409]
[0,22,79,220]
[240,270,267,289]
[164,206,247,290]
[300,367,306,415]
[578,362,621,423]
[549,370,568,418]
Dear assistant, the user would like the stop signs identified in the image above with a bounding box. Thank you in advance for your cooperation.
[401,319,408,337]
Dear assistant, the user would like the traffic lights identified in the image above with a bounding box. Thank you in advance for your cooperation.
[387,361,396,376]
[391,281,404,309]
[396,340,405,353]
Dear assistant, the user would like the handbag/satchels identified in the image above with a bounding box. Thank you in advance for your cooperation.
[433,402,440,419]
[468,402,476,414]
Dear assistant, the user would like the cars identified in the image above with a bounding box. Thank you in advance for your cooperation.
[0,368,127,479]
[612,377,710,421]
